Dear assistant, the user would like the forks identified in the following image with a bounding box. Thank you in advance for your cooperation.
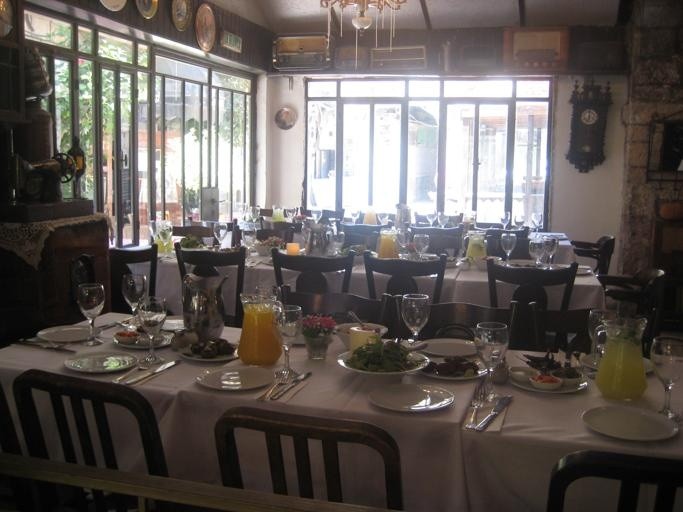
[115,352,155,384]
[256,369,291,401]
[465,383,489,429]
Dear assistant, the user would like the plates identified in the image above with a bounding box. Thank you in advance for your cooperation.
[367,383,456,413]
[36,325,104,342]
[120,318,143,327]
[113,332,176,349]
[63,350,139,375]
[337,350,430,375]
[178,347,240,361]
[580,405,679,443]
[419,361,488,381]
[582,353,654,374]
[160,319,186,332]
[197,368,272,391]
[508,367,588,394]
[421,338,478,358]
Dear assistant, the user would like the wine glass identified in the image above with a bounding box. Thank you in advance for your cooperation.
[77,283,106,346]
[474,322,510,409]
[401,293,430,345]
[142,203,593,281]
[651,336,682,422]
[279,304,303,378]
[121,274,147,325]
[138,295,168,371]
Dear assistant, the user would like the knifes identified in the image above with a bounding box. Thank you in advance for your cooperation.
[270,372,311,400]
[475,396,513,432]
[126,359,180,385]
[10,340,78,354]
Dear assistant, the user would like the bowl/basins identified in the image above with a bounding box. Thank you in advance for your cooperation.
[334,322,390,350]
[530,376,562,391]
[552,368,583,387]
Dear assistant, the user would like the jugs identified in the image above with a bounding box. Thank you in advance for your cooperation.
[179,265,230,343]
[592,317,649,402]
[237,292,286,368]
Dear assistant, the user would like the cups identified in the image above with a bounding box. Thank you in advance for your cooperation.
[301,332,331,360]
[256,282,282,303]
[587,309,617,365]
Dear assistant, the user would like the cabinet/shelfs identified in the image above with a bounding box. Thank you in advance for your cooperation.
[0,0,32,126]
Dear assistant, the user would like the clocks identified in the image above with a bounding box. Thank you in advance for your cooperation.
[565,78,614,173]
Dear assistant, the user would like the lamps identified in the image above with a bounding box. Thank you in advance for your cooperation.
[319,0,409,71]
